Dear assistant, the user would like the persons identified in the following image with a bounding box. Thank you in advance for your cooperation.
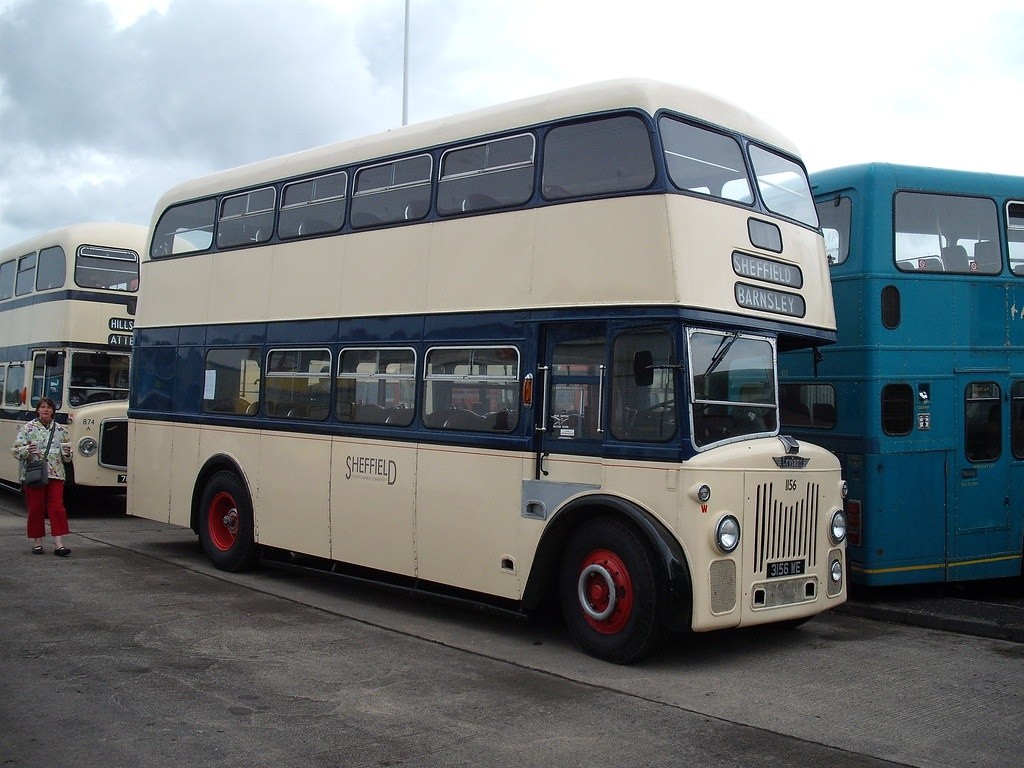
[12,396,72,555]
[309,366,331,392]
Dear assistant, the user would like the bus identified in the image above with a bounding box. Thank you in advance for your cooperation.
[0,220,200,521]
[713,163,1022,591]
[127,76,851,667]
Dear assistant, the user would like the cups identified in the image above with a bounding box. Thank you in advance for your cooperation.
[60,442,71,456]
[30,443,40,454]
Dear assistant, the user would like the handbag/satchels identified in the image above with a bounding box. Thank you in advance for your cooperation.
[25,459,49,488]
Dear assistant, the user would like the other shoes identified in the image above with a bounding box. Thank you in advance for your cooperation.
[54,547,71,556]
[32,546,43,554]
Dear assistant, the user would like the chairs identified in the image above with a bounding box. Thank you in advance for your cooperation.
[288,404,325,420]
[482,412,516,430]
[255,225,291,242]
[974,240,1000,272]
[212,396,250,413]
[620,388,651,434]
[385,409,426,425]
[217,230,250,247]
[355,404,384,423]
[298,219,335,236]
[351,212,383,226]
[918,258,943,271]
[443,410,483,431]
[461,193,500,211]
[245,400,279,416]
[404,200,446,220]
[427,410,448,427]
